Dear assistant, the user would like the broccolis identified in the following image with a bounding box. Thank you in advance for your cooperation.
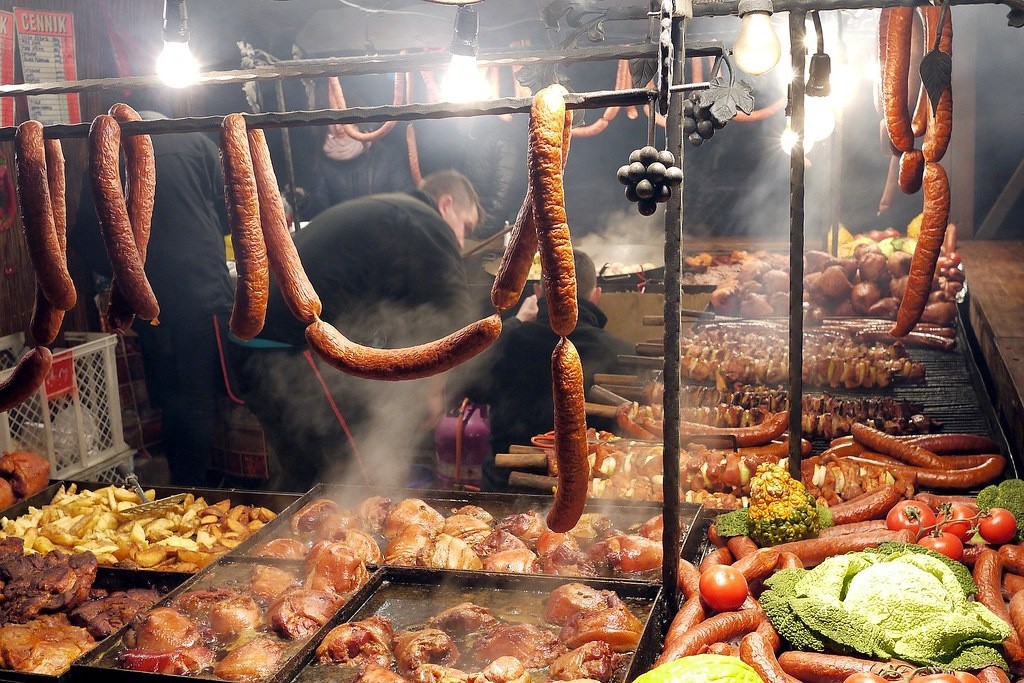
[975,479,1024,543]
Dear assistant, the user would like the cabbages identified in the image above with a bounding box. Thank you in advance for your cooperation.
[757,543,1011,676]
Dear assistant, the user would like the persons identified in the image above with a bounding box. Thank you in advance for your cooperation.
[73,90,231,488]
[228,171,480,490]
[465,128,519,236]
[285,90,406,221]
[468,249,643,493]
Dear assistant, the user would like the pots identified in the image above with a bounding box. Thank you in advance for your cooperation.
[483,244,686,292]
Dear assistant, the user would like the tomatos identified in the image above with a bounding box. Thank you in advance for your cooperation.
[976,508,1018,544]
[917,532,963,563]
[935,506,979,543]
[699,564,748,612]
[886,500,937,540]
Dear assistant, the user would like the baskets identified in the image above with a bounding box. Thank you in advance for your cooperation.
[66,449,136,483]
[0,328,130,481]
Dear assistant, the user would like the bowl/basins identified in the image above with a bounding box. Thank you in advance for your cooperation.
[460,239,484,269]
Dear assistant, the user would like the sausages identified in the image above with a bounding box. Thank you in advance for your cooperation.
[614,485,1024,683]
[0,42,786,534]
[697,5,964,350]
[617,407,1004,490]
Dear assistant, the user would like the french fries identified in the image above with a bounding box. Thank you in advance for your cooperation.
[0,483,150,557]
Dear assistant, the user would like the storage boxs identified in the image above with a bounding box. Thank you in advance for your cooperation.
[0,332,138,484]
